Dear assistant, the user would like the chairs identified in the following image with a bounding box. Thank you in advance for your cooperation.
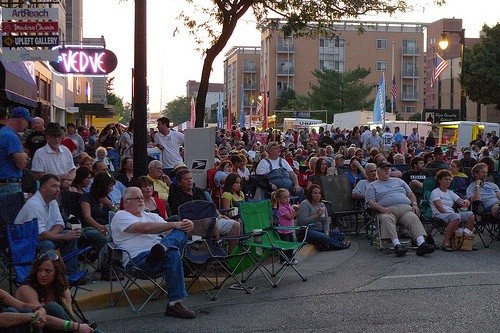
[0,198,311,323]
[321,174,499,248]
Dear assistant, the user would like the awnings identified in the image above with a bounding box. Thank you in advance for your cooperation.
[0,33,38,108]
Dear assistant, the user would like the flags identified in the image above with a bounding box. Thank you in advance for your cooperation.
[217,80,268,129]
[373,75,385,124]
[390,74,397,99]
[190,98,195,128]
[431,46,449,88]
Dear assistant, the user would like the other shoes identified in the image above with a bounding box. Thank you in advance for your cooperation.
[145,244,165,266]
[100,269,125,282]
[394,245,408,256]
[442,245,453,252]
[291,259,298,265]
[319,245,329,251]
[416,242,435,256]
[280,261,289,265]
[472,246,478,251]
[344,241,351,248]
[165,302,196,319]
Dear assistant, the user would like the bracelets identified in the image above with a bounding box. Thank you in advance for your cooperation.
[67,320,72,330]
[64,321,69,332]
[475,185,479,188]
[75,322,80,331]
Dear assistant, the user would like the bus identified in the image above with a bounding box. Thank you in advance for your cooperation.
[283,117,323,132]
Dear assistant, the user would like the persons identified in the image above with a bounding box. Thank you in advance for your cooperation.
[466,163,500,241]
[365,162,435,257]
[154,117,185,179]
[0,107,500,333]
[14,174,90,286]
[168,170,241,254]
[271,188,301,265]
[429,169,478,252]
[296,184,350,250]
[110,187,196,319]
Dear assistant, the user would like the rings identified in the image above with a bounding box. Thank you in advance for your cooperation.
[38,321,40,323]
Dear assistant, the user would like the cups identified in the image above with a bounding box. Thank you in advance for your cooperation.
[192,235,202,248]
[318,208,326,217]
[72,224,81,237]
[254,229,262,241]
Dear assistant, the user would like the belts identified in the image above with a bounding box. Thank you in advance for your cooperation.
[0,178,23,183]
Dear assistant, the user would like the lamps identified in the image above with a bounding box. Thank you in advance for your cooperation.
[258,89,270,100]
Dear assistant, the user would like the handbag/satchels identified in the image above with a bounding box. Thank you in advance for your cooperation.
[450,232,473,251]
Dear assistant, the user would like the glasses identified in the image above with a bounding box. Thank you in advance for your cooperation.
[47,135,62,139]
[104,173,111,186]
[38,252,60,263]
[366,169,376,175]
[126,196,145,201]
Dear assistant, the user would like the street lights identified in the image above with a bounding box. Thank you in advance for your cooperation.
[438,29,465,121]
[258,91,270,116]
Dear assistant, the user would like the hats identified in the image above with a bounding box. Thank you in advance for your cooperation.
[42,122,64,135]
[430,147,446,154]
[172,162,188,172]
[462,147,471,154]
[335,154,346,160]
[376,161,391,167]
[75,166,95,183]
[10,107,35,122]
